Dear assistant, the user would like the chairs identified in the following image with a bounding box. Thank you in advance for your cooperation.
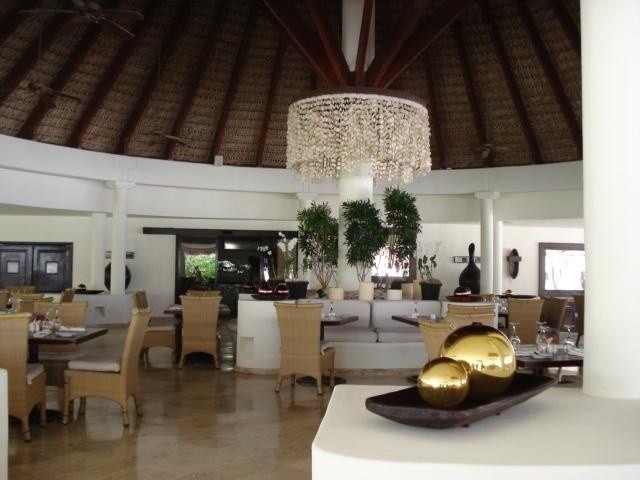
[273,301,335,396]
[418,294,584,376]
[0,286,232,442]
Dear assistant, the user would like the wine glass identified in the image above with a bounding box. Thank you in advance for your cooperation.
[535,321,551,355]
[562,324,576,357]
[508,321,521,353]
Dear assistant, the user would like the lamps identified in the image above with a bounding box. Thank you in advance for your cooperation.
[262,0,477,185]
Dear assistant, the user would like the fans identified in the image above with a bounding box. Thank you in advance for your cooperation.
[18,0,144,39]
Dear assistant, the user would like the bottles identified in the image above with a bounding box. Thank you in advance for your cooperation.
[411,302,418,319]
[328,301,335,319]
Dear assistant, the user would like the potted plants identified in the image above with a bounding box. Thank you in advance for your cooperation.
[257,186,443,301]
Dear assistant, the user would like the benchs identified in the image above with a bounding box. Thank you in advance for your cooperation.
[294,300,498,370]
